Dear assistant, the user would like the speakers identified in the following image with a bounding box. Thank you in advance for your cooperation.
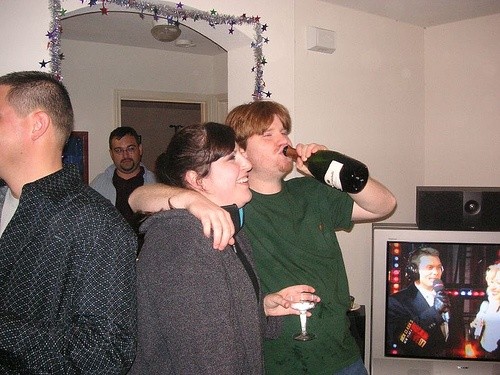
[416,186,500,231]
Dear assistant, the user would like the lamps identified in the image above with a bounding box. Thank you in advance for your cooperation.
[150,17,182,43]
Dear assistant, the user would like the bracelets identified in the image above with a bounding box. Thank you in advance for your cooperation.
[168,194,175,209]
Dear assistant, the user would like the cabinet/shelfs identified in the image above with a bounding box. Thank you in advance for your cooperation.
[348,305,365,365]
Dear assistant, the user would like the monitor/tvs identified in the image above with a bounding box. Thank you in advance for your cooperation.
[371,222,500,375]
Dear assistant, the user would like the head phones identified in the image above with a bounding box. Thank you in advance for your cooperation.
[403,247,444,281]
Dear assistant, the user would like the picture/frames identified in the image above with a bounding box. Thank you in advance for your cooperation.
[62,131,88,186]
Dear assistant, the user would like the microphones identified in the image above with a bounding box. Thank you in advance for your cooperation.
[474,301,488,340]
[433,279,446,313]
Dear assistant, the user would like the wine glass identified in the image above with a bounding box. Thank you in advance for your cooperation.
[288,285,318,342]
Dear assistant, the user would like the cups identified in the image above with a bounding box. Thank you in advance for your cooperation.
[348,294,355,308]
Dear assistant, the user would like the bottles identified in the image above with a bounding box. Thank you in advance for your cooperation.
[283,144,369,194]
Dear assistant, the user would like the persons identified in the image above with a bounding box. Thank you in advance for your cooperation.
[467,263,500,361]
[127,99,397,375]
[1,71,139,374]
[387,247,461,359]
[88,126,157,257]
[124,122,321,375]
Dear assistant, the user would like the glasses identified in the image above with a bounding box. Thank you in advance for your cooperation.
[422,265,444,273]
[114,147,136,153]
